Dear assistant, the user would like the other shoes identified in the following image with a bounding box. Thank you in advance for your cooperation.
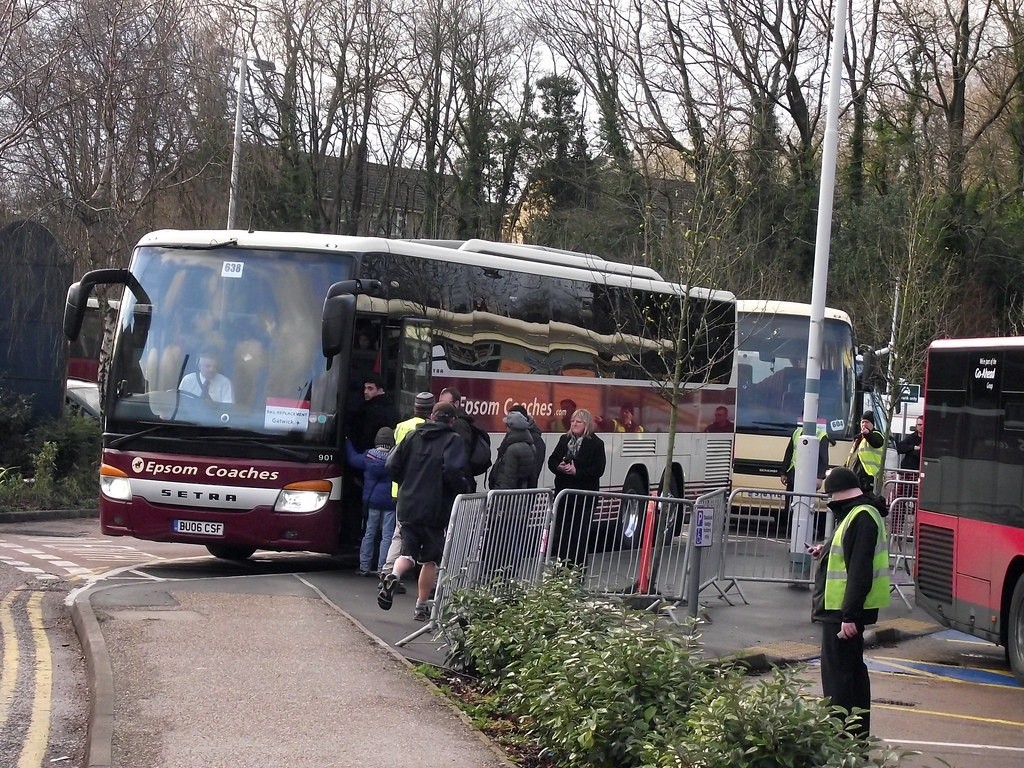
[412,607,427,622]
[355,568,369,577]
[378,570,389,589]
[395,582,406,594]
[377,573,398,611]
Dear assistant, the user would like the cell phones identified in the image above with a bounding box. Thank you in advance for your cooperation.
[804,543,821,555]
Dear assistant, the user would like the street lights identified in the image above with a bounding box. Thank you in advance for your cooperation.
[214,47,276,230]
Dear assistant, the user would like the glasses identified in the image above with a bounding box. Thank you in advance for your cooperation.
[918,424,922,426]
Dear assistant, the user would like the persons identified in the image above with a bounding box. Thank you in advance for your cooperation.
[346,373,473,622]
[780,410,829,537]
[488,405,546,578]
[844,412,885,493]
[748,355,805,407]
[549,399,577,432]
[548,409,606,582]
[179,353,234,403]
[892,415,923,541]
[594,403,646,433]
[807,467,891,760]
[705,406,734,432]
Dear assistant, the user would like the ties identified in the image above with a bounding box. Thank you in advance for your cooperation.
[200,379,210,400]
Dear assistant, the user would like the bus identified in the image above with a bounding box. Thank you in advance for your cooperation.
[65,228,738,560]
[913,336,1024,686]
[728,300,877,535]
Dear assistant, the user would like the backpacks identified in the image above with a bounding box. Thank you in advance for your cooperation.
[457,411,493,490]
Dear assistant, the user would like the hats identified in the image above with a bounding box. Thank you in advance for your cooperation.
[861,410,874,426]
[414,391,437,408]
[824,467,860,493]
[374,426,395,448]
[622,401,634,416]
[431,400,458,419]
[508,405,528,417]
[502,411,530,431]
